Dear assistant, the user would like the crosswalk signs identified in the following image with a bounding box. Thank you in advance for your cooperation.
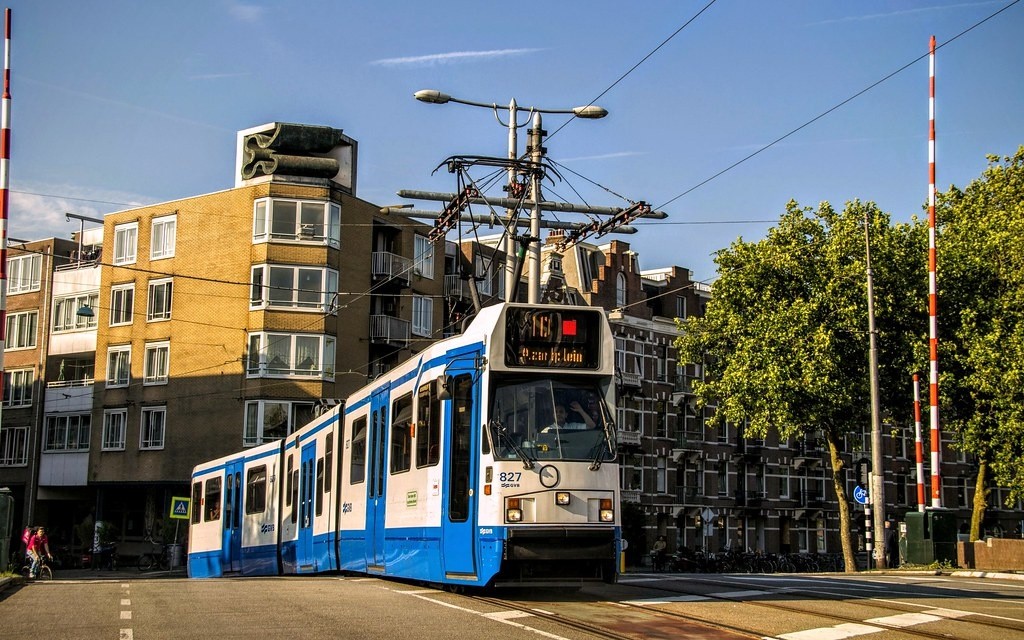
[170,497,191,520]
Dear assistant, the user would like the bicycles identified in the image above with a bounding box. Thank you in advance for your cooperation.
[650,546,860,573]
[21,555,53,580]
[137,538,171,571]
[50,546,78,569]
[97,541,119,572]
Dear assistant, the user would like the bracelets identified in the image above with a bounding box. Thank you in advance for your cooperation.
[47,552,50,554]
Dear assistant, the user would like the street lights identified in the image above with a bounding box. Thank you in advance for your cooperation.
[414,88,609,300]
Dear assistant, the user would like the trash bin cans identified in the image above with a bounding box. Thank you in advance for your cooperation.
[905,509,958,566]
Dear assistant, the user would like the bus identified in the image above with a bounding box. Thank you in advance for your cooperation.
[188,301,621,599]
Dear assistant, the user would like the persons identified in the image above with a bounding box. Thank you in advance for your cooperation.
[209,499,220,521]
[26,527,53,579]
[653,536,667,572]
[540,400,596,434]
